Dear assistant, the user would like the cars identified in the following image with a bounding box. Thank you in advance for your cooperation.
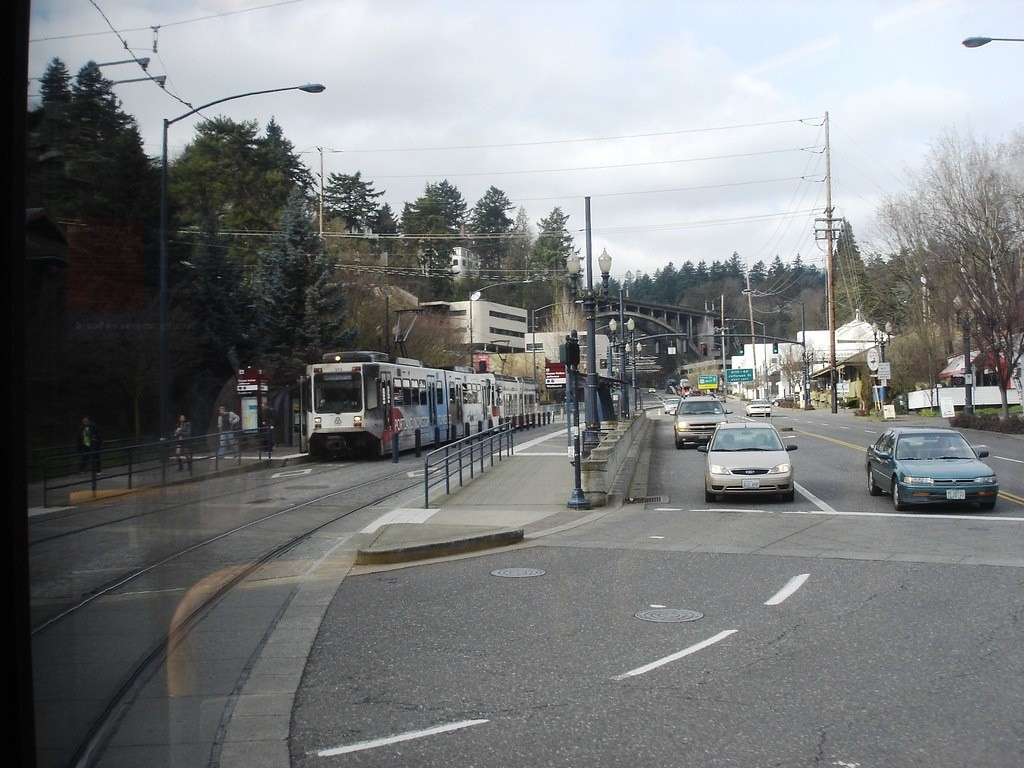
[746,395,800,418]
[697,423,798,503]
[865,427,999,513]
[648,388,727,414]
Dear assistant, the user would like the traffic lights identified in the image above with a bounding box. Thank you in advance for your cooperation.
[560,343,580,365]
[738,343,744,356]
[701,343,707,356]
[773,342,778,354]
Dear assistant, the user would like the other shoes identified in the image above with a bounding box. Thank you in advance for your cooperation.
[218,455,224,459]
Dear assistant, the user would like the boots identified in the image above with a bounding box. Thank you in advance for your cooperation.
[177,459,184,472]
[187,459,191,471]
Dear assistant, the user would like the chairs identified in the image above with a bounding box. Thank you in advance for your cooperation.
[753,434,775,447]
[716,434,738,448]
[897,442,916,458]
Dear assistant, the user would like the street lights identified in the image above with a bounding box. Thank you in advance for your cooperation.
[625,342,642,388]
[725,319,768,397]
[567,245,612,453]
[609,317,635,416]
[872,322,893,363]
[160,83,326,461]
[953,294,980,416]
[469,280,533,374]
[743,289,811,407]
[532,300,583,380]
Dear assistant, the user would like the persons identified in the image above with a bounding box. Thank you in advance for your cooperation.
[174,415,193,472]
[261,403,277,454]
[76,414,103,477]
[930,437,960,458]
[217,406,240,460]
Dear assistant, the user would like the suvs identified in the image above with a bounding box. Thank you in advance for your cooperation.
[669,394,729,449]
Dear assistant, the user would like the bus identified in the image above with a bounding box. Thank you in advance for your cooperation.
[304,350,538,463]
